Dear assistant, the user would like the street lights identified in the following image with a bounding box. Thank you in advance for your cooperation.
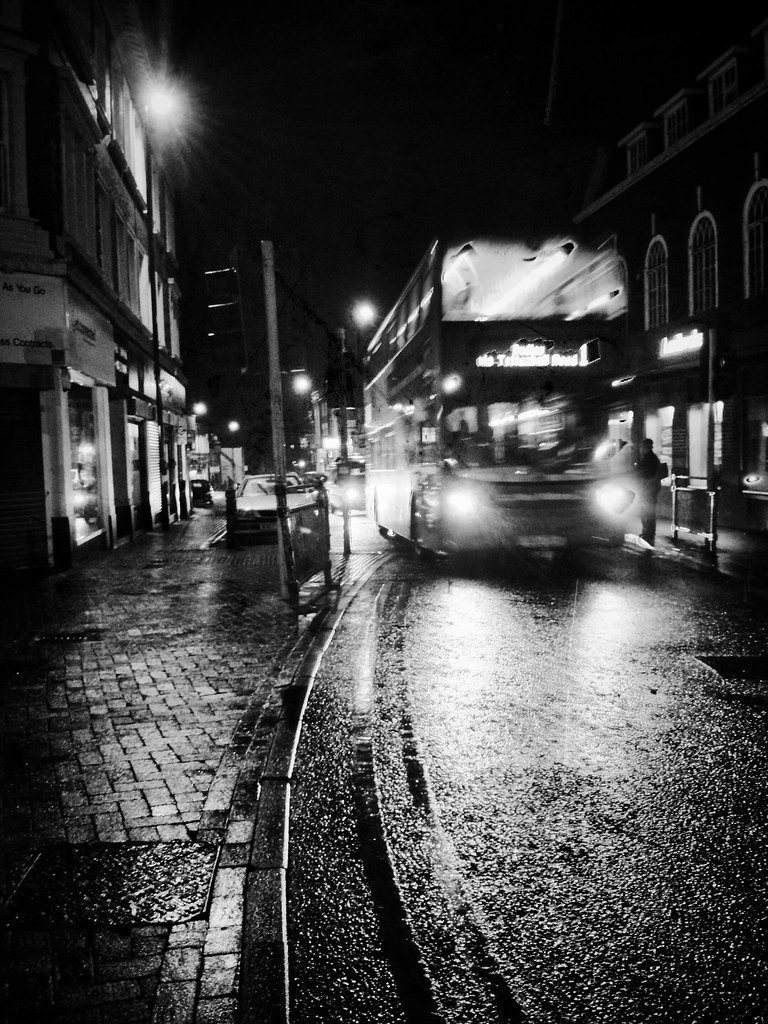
[193,403,207,472]
[229,421,239,484]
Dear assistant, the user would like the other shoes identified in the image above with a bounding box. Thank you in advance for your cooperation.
[644,537,655,546]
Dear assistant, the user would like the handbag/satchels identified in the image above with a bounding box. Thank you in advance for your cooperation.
[653,462,668,481]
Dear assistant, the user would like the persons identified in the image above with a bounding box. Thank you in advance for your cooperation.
[635,438,663,540]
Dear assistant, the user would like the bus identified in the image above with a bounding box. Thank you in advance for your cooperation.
[363,236,631,553]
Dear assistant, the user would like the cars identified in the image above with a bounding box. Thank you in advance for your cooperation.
[233,470,314,536]
[326,457,366,514]
